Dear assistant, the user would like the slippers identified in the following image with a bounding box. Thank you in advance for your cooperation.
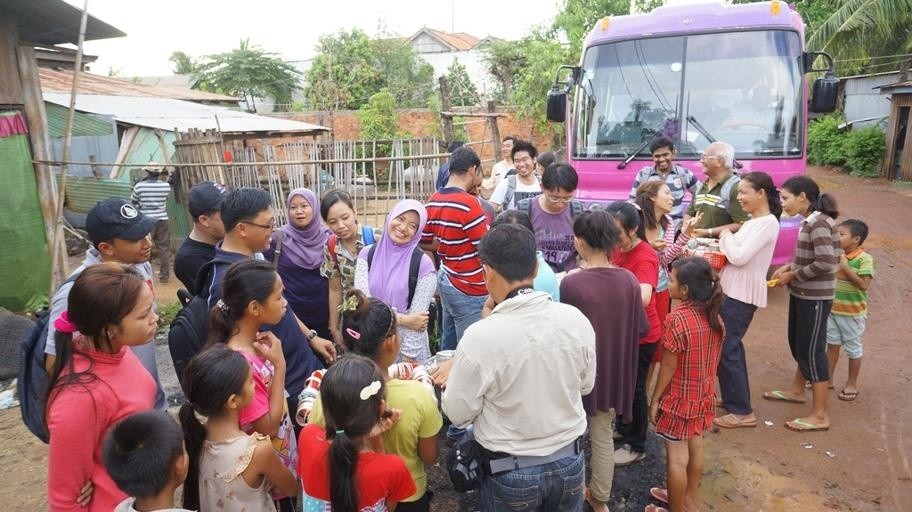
[586,487,610,512]
[838,389,859,401]
[784,418,829,432]
[764,390,805,404]
[645,502,669,512]
[713,413,756,429]
[805,379,834,390]
[716,398,724,408]
[650,487,669,505]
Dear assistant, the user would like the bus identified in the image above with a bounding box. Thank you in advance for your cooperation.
[544,1,840,282]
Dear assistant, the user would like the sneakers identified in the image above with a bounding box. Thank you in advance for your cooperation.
[613,443,646,467]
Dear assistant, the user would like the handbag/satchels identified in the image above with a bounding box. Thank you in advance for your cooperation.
[445,441,486,493]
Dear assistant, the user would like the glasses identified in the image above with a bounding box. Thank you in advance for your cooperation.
[544,196,576,204]
[700,154,721,162]
[233,217,277,231]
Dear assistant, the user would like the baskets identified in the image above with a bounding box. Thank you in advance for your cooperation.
[684,249,726,271]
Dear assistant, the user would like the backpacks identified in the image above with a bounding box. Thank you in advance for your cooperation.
[16,272,82,444]
[167,257,236,386]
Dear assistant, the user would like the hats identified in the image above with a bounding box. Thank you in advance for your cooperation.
[86,198,160,240]
[143,162,162,173]
[188,181,233,218]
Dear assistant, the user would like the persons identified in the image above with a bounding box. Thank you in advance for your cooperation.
[102,410,191,512]
[711,171,782,427]
[46,263,156,512]
[806,218,875,401]
[733,86,778,132]
[131,161,172,283]
[44,197,165,411]
[763,176,842,432]
[175,133,750,510]
